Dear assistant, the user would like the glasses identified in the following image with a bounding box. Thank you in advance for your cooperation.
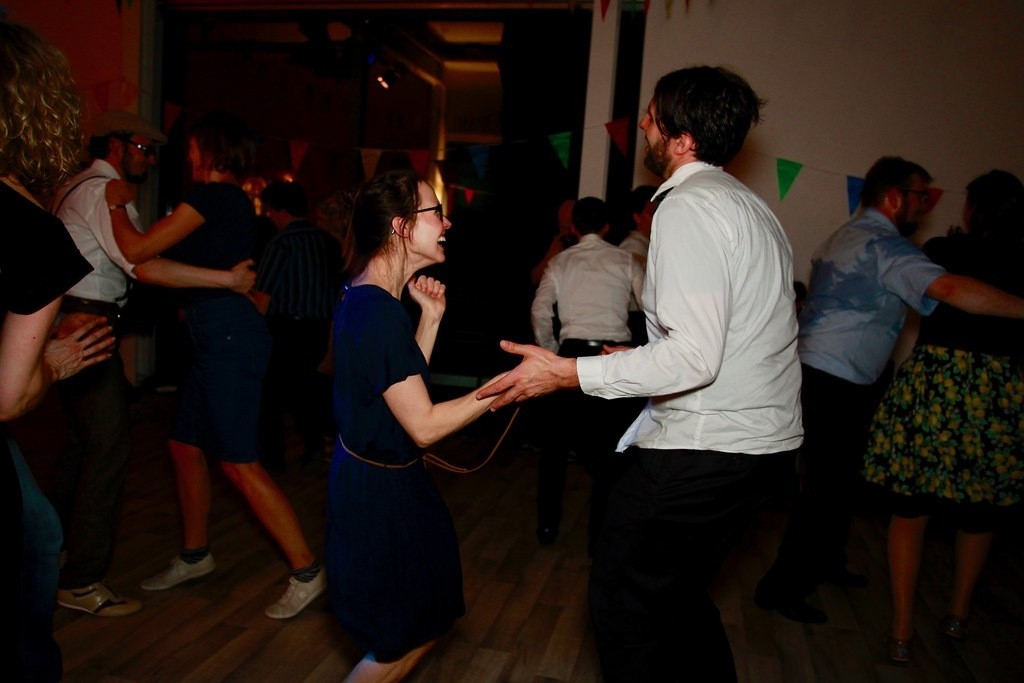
[908,189,931,201]
[414,204,445,222]
[127,139,157,158]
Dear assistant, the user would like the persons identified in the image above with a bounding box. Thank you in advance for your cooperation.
[531,195,649,539]
[749,156,1024,628]
[529,199,596,359]
[861,167,1023,668]
[0,17,398,682]
[477,64,804,683]
[323,166,529,683]
[608,184,666,474]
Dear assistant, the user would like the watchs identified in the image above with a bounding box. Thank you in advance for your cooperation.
[109,204,126,214]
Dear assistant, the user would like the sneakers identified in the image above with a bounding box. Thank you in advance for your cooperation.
[266,565,324,620]
[141,552,213,590]
[56,582,142,618]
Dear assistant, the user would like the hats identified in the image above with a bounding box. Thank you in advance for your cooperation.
[86,110,168,143]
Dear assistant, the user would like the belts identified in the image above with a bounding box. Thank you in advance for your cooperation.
[563,339,631,348]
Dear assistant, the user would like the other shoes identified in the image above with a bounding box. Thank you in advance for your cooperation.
[750,585,825,623]
[828,570,868,591]
[539,522,558,544]
[885,637,913,665]
[944,613,977,637]
